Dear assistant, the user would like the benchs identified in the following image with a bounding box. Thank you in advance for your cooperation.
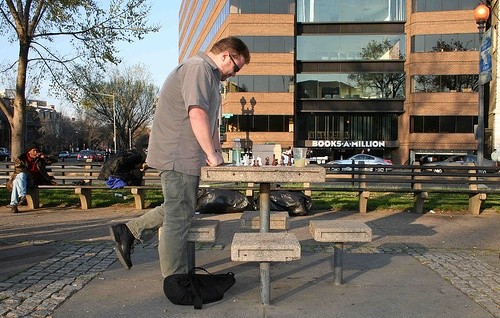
[0,163,500,215]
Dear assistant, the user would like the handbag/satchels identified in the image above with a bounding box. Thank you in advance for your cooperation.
[163,267,236,309]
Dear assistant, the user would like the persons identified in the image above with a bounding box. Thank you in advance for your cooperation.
[104,148,111,161]
[10,142,58,213]
[491,149,498,161]
[110,37,251,278]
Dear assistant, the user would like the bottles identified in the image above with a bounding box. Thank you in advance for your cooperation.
[233,139,241,165]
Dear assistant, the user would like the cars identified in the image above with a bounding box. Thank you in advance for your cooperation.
[0,148,11,162]
[326,154,393,173]
[422,154,500,174]
[59,151,69,158]
[281,153,294,164]
[76,150,98,163]
[95,151,104,162]
[70,152,78,158]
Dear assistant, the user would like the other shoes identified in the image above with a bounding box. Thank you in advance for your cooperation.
[12,206,18,213]
[19,198,27,206]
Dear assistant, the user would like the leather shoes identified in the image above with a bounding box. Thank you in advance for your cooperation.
[109,224,133,270]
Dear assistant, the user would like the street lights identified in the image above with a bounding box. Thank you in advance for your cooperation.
[219,80,228,143]
[474,0,491,189]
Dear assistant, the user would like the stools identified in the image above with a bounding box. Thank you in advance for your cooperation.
[158,220,221,275]
[240,211,289,230]
[309,219,372,285]
[230,233,301,305]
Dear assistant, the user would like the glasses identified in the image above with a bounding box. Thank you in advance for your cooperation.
[229,54,241,73]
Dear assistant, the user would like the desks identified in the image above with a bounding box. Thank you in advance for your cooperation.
[200,164,326,233]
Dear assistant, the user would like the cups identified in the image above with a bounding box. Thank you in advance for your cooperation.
[293,148,308,168]
[244,156,249,165]
